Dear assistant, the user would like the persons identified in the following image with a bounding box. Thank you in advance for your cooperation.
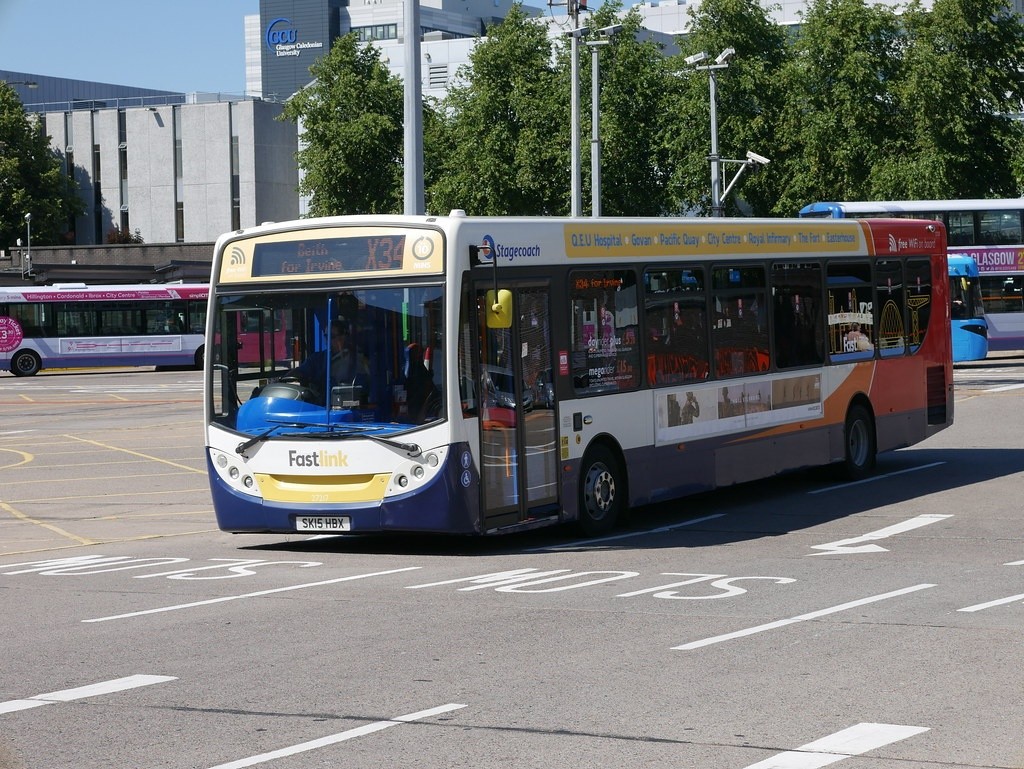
[285,320,370,407]
[600,304,612,349]
[847,322,866,341]
[668,392,700,427]
[720,387,730,418]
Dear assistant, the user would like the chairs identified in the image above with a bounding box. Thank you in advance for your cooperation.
[948,230,1022,247]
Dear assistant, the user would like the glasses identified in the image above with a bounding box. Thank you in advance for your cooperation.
[324,334,344,340]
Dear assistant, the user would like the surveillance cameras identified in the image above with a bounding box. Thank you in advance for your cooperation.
[597,24,624,38]
[684,51,707,64]
[746,151,771,164]
[714,48,735,65]
[565,27,590,38]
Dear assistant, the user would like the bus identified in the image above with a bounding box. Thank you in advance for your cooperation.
[202,209,954,540]
[0,282,291,377]
[645,196,1024,362]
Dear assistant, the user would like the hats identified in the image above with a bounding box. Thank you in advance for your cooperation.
[687,392,693,399]
[722,387,728,395]
